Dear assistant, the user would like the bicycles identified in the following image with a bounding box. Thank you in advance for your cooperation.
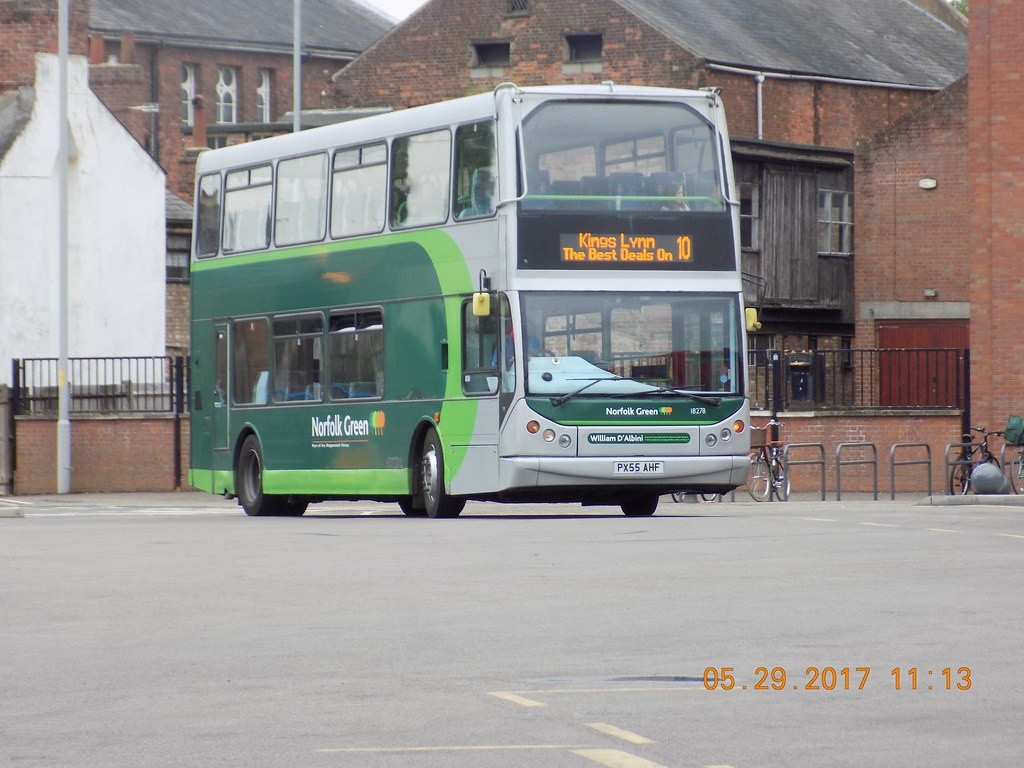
[950,427,1006,496]
[671,490,718,504]
[1010,443,1024,495]
[746,420,791,503]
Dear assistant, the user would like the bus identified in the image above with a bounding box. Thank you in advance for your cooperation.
[186,81,768,519]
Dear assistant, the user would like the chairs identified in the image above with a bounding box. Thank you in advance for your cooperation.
[392,167,723,227]
[274,382,374,404]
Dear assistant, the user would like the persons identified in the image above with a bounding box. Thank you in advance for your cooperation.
[662,181,689,211]
[491,318,539,366]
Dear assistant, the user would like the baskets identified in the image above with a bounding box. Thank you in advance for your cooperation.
[750,428,766,448]
